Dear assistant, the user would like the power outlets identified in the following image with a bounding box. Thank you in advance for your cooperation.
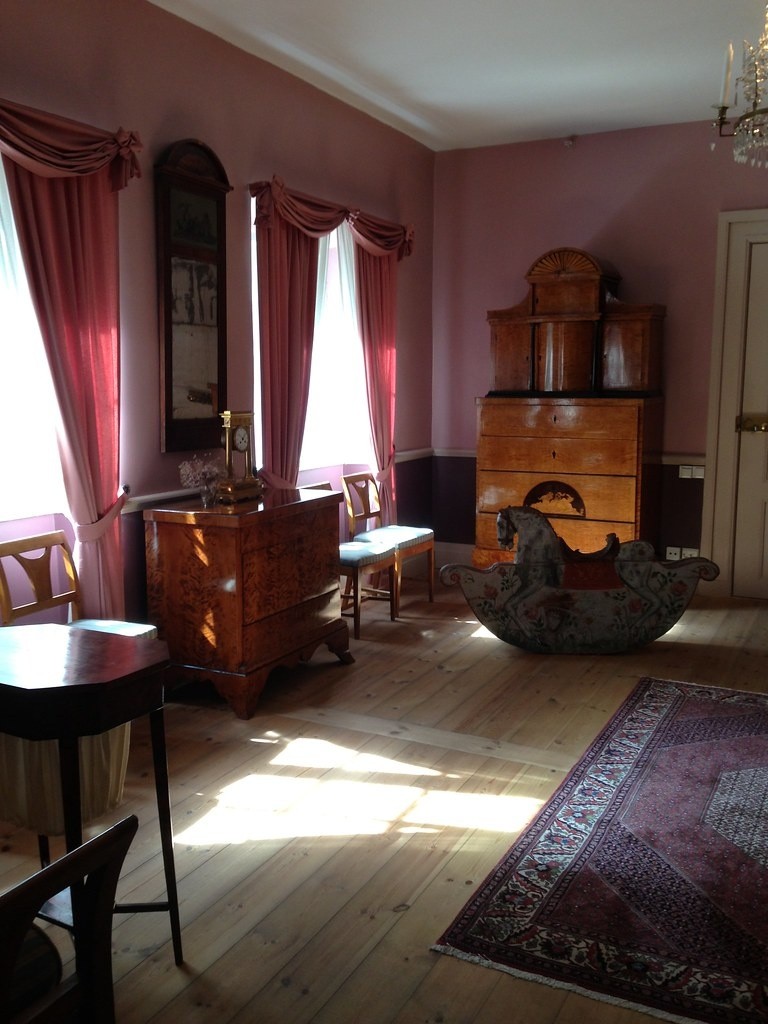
[666,547,681,560]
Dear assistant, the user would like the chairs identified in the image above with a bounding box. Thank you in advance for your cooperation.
[342,471,435,617]
[0,816,139,1024]
[298,482,395,640]
[0,530,157,641]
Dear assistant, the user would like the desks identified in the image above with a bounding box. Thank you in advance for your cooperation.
[0,623,184,970]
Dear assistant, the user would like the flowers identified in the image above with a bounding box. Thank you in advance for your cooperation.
[179,453,227,487]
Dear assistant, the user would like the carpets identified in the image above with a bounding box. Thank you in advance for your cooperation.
[430,676,768,1024]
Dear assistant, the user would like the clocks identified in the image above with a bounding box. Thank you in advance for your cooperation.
[215,409,267,505]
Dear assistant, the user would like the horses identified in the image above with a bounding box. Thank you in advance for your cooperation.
[497,505,663,640]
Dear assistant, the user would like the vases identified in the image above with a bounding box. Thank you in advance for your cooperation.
[200,486,214,508]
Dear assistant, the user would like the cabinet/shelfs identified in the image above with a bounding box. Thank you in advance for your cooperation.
[473,246,667,571]
[143,489,356,720]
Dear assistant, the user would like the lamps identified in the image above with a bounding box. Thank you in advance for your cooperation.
[710,3,768,168]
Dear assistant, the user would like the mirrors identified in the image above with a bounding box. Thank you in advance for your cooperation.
[153,139,233,453]
